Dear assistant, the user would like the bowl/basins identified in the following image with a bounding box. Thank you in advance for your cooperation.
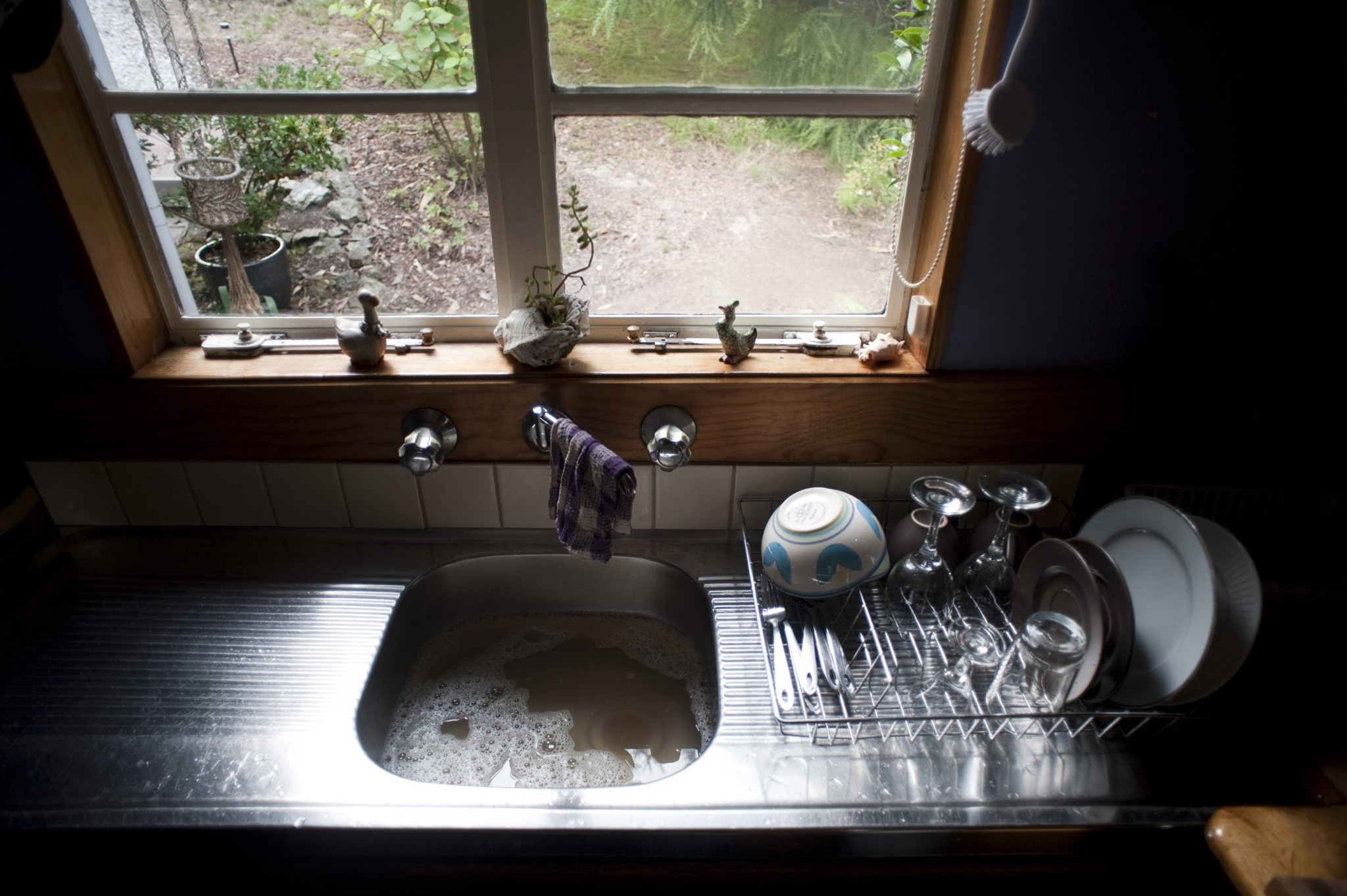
[761,487,891,599]
[967,507,1040,571]
[887,507,964,571]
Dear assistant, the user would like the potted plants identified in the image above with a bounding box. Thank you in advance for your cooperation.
[195,50,369,312]
[129,113,245,226]
[494,184,597,368]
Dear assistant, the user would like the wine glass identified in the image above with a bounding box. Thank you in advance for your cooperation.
[950,469,1051,627]
[907,616,1006,734]
[884,475,977,632]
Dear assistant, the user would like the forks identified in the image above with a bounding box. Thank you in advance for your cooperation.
[760,574,856,711]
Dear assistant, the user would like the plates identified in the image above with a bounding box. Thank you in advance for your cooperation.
[1077,495,1262,707]
[1012,537,1134,704]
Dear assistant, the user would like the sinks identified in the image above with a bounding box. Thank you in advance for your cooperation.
[354,549,725,793]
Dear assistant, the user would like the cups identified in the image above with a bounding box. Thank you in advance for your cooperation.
[986,610,1087,737]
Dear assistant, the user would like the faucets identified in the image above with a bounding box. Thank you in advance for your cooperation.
[523,405,638,499]
